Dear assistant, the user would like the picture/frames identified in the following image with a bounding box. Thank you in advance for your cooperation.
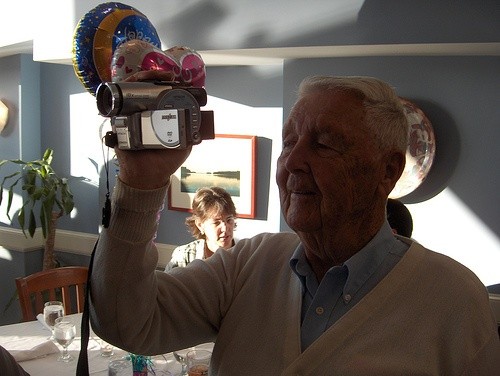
[168,134,257,219]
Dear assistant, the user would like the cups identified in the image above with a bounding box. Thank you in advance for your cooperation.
[187,350,212,376]
[108,365,118,376]
[100,340,113,357]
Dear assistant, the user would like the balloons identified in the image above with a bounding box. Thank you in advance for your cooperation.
[111,39,206,89]
[73,2,161,96]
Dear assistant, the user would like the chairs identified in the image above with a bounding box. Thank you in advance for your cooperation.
[16,266,89,321]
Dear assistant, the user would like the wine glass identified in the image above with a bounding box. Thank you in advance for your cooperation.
[54,318,76,362]
[174,347,195,376]
[44,301,64,340]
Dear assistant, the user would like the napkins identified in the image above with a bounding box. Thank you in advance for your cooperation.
[7,336,64,362]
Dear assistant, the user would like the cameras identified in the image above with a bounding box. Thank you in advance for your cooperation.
[95,81,214,153]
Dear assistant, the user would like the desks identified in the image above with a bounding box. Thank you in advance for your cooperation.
[0,313,214,376]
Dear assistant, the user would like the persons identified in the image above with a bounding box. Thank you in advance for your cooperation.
[387,198,413,238]
[88,72,500,376]
[164,187,237,272]
